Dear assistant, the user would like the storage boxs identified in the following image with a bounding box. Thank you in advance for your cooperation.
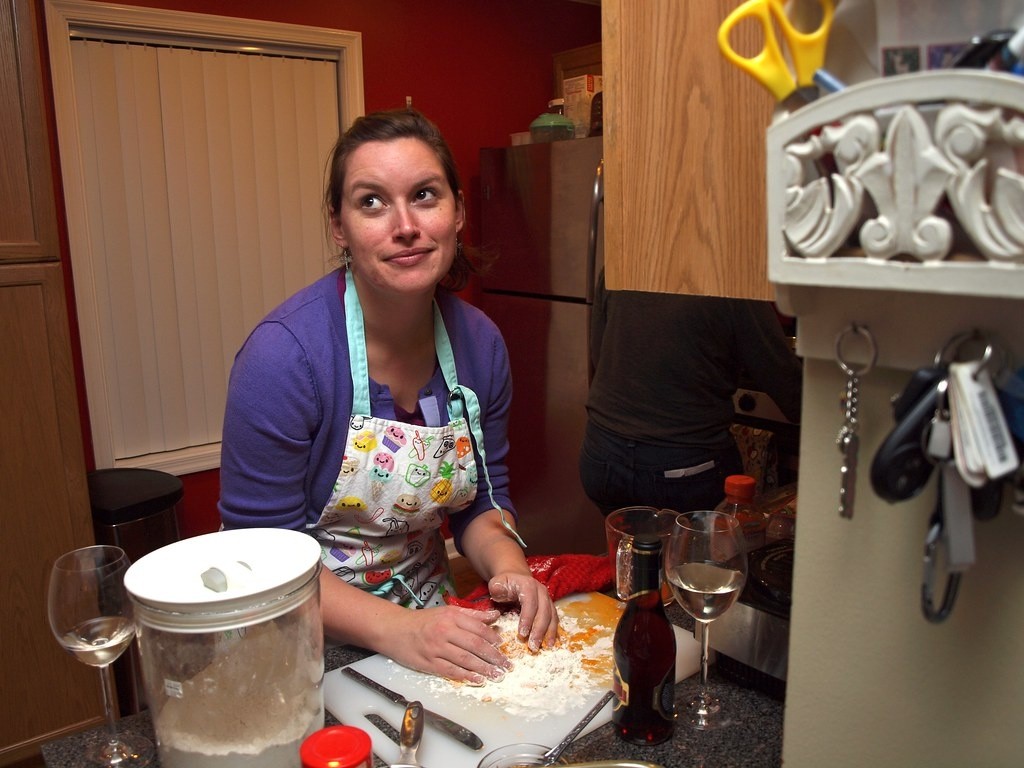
[564,75,603,138]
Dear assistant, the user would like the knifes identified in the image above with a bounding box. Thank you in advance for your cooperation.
[341,666,484,751]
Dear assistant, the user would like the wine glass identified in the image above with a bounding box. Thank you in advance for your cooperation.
[666,511,748,730]
[49,546,154,768]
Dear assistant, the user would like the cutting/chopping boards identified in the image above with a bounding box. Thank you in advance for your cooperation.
[323,588,714,768]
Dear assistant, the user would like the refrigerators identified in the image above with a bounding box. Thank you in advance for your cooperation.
[466,137,601,553]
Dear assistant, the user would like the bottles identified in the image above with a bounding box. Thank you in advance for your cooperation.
[612,535,677,745]
[709,475,769,573]
[124,525,323,768]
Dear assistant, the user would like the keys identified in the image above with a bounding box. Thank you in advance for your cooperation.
[838,356,1024,519]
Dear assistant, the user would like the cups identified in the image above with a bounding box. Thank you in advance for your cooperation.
[477,743,569,768]
[607,507,690,607]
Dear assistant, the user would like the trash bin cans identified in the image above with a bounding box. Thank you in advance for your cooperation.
[87,467,187,715]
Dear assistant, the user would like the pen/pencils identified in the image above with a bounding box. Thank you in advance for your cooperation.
[813,67,846,93]
[967,23,1024,71]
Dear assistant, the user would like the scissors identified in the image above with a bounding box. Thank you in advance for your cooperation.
[717,0,837,177]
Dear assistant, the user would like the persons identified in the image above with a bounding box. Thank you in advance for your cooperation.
[578,266,803,536]
[217,109,558,684]
